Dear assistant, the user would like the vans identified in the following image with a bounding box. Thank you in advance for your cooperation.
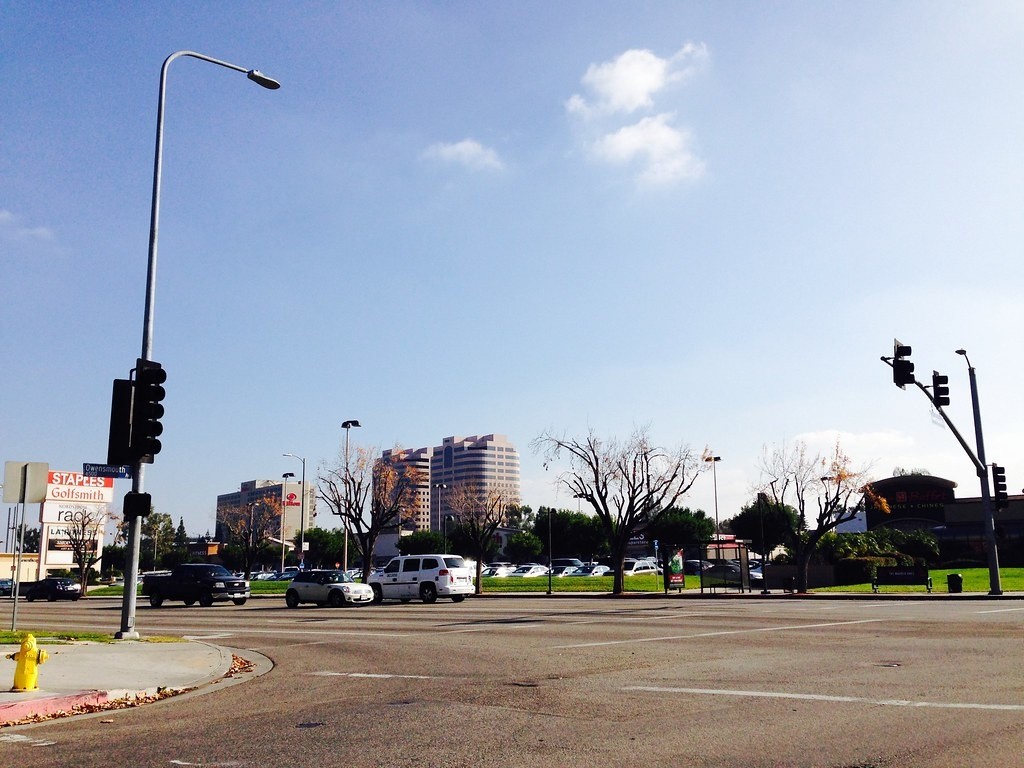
[366,554,476,604]
[549,558,585,567]
[284,566,300,573]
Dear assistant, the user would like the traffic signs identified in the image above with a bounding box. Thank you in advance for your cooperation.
[83,464,133,478]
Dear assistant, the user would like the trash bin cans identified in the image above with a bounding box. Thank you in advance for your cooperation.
[947,574,962,593]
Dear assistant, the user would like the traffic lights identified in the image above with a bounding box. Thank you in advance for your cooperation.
[131,358,167,464]
[993,462,1008,511]
[894,339,916,388]
[934,370,949,411]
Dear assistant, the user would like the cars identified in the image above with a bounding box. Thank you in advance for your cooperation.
[26,577,82,602]
[482,562,611,578]
[347,567,385,580]
[0,579,16,595]
[683,558,763,581]
[623,556,664,576]
[101,577,116,585]
[233,571,298,581]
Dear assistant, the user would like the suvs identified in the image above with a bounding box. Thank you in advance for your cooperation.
[286,569,375,608]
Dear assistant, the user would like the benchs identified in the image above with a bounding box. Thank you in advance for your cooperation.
[872,565,933,593]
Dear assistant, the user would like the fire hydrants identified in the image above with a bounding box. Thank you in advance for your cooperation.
[7,634,49,693]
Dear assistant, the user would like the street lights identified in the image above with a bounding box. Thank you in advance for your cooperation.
[282,472,295,574]
[433,483,455,555]
[246,502,259,547]
[116,47,280,640]
[284,453,304,573]
[341,420,361,572]
[955,350,1002,596]
[547,507,556,593]
[705,456,721,560]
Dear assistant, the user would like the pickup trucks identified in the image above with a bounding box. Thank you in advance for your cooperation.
[142,563,250,606]
